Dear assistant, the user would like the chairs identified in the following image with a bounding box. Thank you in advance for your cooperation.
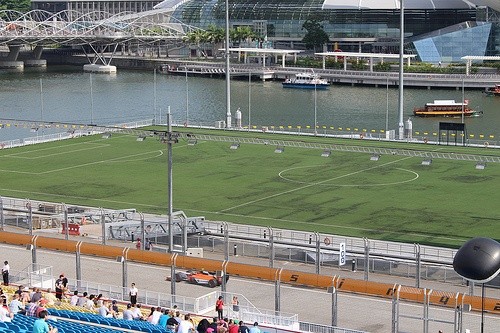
[0,284,174,333]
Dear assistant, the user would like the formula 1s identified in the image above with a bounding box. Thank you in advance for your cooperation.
[175,267,227,288]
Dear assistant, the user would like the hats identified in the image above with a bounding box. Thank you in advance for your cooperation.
[23,287,29,291]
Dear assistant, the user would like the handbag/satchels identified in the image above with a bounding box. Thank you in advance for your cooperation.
[216,305,218,311]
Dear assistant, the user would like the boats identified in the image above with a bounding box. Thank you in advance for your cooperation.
[414,99,480,118]
[281,68,331,90]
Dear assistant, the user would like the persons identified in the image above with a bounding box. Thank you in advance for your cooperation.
[147,305,261,333]
[1,261,10,286]
[145,237,153,250]
[71,290,119,319]
[231,296,240,318]
[123,303,143,321]
[136,238,141,249]
[0,275,70,333]
[128,283,139,304]
[214,296,228,319]
